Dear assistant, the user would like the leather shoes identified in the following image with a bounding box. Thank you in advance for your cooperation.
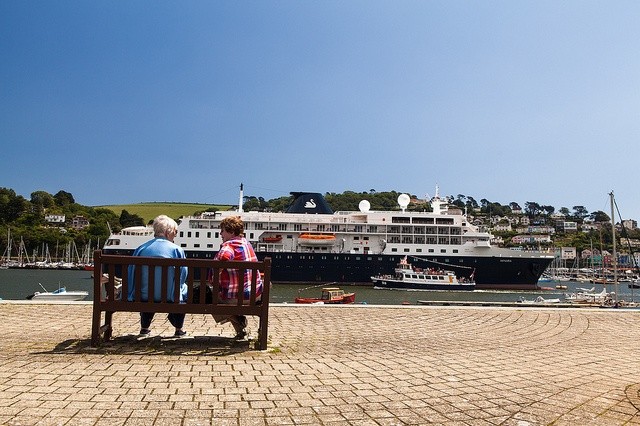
[175,330,188,337]
[139,328,153,337]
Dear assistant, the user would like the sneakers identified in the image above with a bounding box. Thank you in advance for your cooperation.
[234,330,246,338]
[236,315,248,328]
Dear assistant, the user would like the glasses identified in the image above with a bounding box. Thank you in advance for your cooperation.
[172,229,178,233]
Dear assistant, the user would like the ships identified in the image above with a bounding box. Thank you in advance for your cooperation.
[102,182,556,290]
[370,255,476,291]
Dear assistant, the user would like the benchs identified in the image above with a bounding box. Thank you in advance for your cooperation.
[92,249,270,349]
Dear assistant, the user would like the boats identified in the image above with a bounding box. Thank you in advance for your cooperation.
[565,289,640,308]
[538,269,552,282]
[554,267,640,289]
[30,282,89,301]
[518,296,559,303]
[295,288,356,304]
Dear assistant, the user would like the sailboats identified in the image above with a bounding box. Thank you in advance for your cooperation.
[0,228,101,271]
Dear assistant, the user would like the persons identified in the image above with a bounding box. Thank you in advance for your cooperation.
[127,215,188,335]
[208,216,263,339]
[412,265,447,275]
